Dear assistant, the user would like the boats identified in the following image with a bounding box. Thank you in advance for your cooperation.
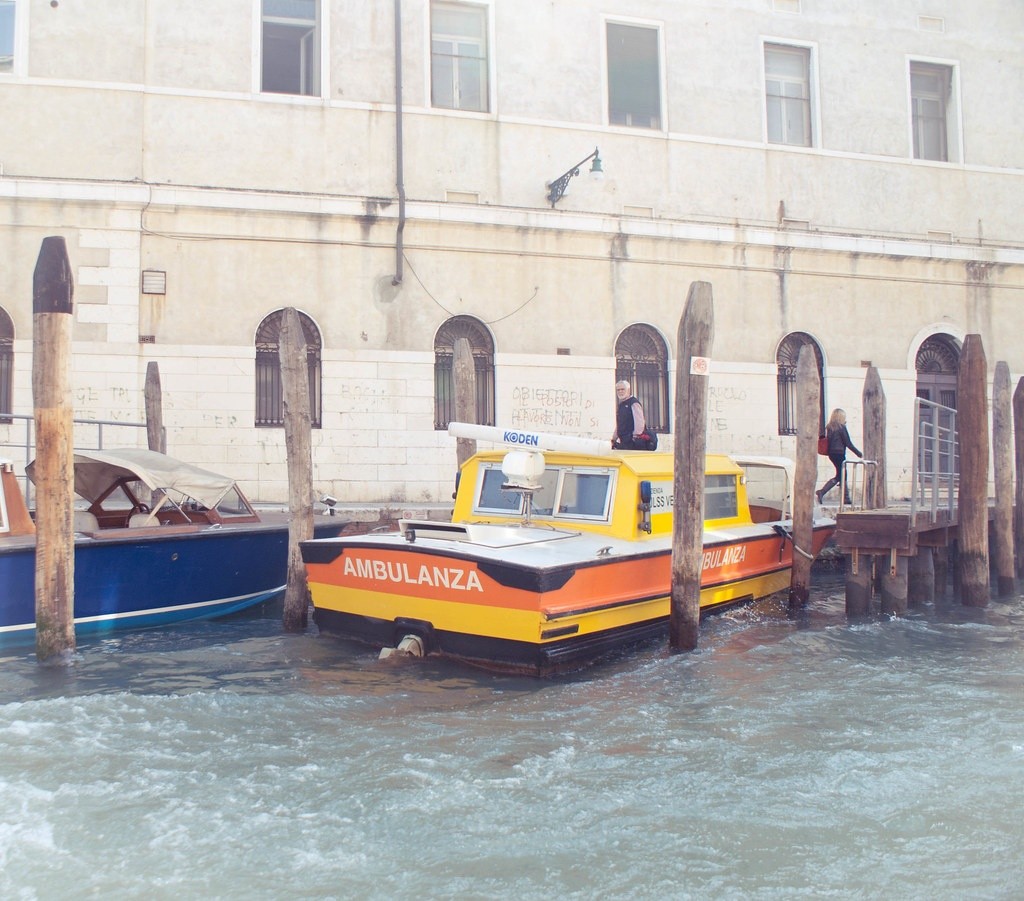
[297,451,836,671]
[1,445,395,653]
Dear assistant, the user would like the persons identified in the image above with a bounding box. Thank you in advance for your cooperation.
[610,380,646,450]
[815,408,862,505]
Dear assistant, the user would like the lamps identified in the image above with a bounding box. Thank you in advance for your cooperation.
[547,148,603,210]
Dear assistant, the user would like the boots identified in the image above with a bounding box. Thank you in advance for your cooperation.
[837,484,852,504]
[816,479,836,504]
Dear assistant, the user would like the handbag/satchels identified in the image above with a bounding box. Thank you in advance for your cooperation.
[818,438,828,455]
[636,430,658,451]
